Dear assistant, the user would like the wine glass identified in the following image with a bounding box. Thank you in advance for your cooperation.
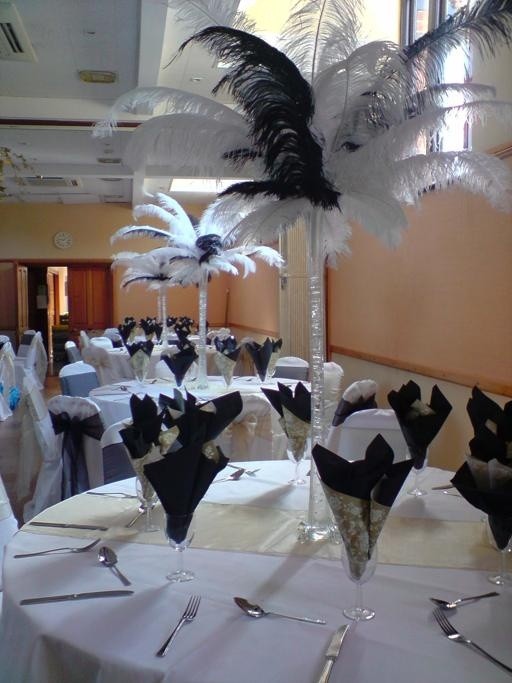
[403,445,429,496]
[285,437,305,486]
[338,540,376,619]
[163,511,198,582]
[134,475,160,531]
[483,517,511,585]
[131,366,278,400]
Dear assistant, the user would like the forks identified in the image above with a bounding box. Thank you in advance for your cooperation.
[432,607,511,672]
[14,537,102,558]
[156,594,202,656]
[226,463,261,474]
[86,491,138,498]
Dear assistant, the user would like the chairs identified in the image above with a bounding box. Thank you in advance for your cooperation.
[2,314,408,503]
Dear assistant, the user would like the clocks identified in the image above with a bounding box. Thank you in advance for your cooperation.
[53,230,75,250]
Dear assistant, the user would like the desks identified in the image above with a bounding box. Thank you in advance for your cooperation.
[1,459,510,681]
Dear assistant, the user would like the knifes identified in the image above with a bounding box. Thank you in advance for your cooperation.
[319,624,349,682]
[30,521,108,530]
[20,589,135,607]
[432,483,453,490]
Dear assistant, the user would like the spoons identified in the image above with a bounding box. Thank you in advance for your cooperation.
[231,596,325,624]
[128,503,157,527]
[98,546,131,586]
[430,591,500,608]
[210,467,247,484]
